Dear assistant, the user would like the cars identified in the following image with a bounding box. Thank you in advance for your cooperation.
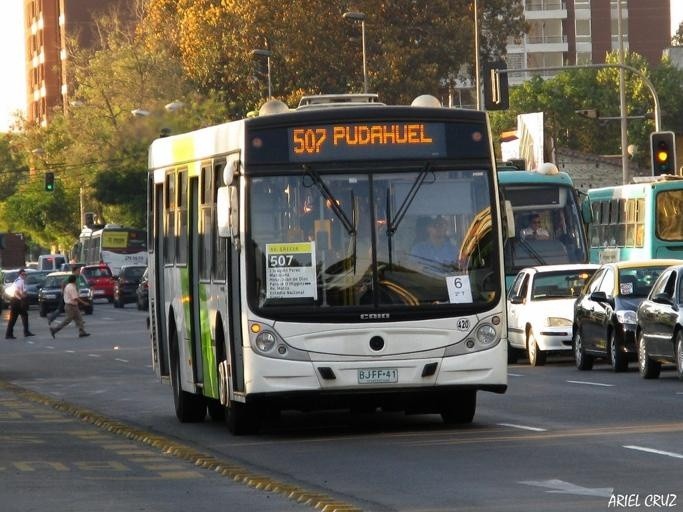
[506,263,683,381]
[1,255,148,317]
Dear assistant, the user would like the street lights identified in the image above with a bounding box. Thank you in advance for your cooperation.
[342,11,370,92]
[252,49,274,100]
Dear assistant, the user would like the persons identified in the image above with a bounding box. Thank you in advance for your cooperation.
[47,274,91,339]
[44,266,86,324]
[4,269,35,339]
[403,216,464,271]
[66,255,77,265]
[521,213,550,240]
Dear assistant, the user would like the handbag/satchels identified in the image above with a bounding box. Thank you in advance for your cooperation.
[5,282,18,300]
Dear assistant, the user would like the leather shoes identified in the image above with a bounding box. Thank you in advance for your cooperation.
[24,333,34,337]
[6,336,16,339]
[79,333,89,337]
[50,327,55,339]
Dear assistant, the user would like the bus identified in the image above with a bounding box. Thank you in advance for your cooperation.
[582,180,682,264]
[146,93,515,435]
[495,163,590,292]
[73,224,148,277]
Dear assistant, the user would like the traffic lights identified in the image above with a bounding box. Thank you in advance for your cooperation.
[651,131,678,174]
[45,173,54,191]
